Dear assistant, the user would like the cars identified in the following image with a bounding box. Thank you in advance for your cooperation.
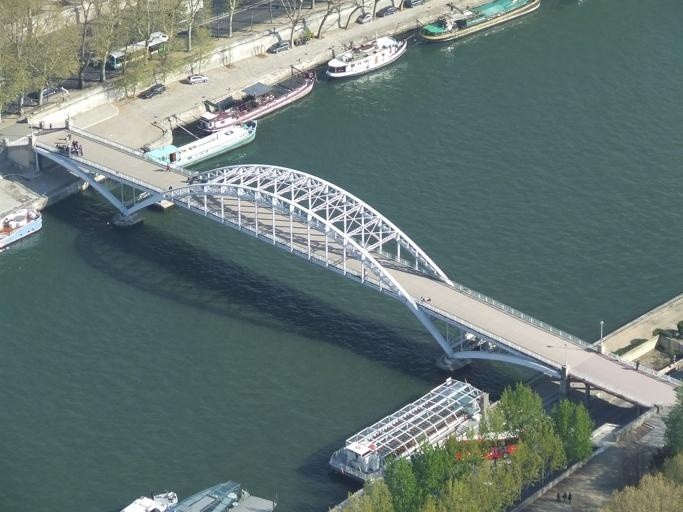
[268,40,289,54]
[357,13,372,24]
[376,6,397,18]
[141,83,166,99]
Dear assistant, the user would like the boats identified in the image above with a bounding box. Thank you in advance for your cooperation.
[418,0,540,42]
[118,489,178,512]
[197,68,316,133]
[0,208,42,248]
[324,36,408,79]
[147,118,258,174]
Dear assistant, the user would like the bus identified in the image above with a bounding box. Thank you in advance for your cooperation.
[451,428,522,463]
[106,31,168,70]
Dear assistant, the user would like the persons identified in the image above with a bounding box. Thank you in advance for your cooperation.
[567,492,572,503]
[561,491,567,503]
[185,175,190,184]
[165,162,171,175]
[168,185,172,190]
[53,132,83,156]
[555,491,561,502]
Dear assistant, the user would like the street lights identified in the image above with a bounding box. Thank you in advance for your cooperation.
[599,319,604,338]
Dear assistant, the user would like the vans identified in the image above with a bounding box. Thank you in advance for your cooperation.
[186,75,207,84]
[405,1,426,9]
